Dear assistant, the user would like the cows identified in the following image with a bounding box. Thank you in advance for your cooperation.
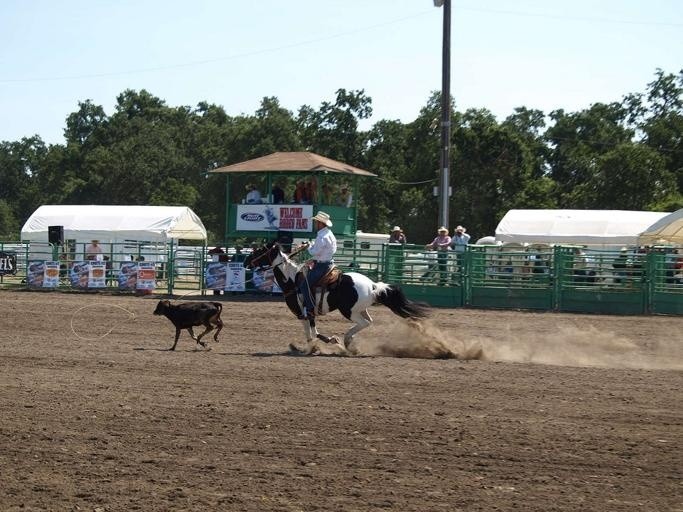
[149,298,224,351]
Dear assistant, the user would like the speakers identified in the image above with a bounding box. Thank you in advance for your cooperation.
[47,226,64,247]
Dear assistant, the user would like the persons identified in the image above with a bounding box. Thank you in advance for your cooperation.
[207,241,268,298]
[388,226,406,284]
[297,211,337,320]
[450,225,471,272]
[424,226,451,286]
[58,238,145,287]
[245,176,353,208]
[525,239,683,290]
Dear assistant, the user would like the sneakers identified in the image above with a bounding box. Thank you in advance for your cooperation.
[299,312,314,320]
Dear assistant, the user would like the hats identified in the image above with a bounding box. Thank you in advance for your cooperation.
[391,226,403,232]
[438,227,448,235]
[246,183,257,190]
[341,185,348,190]
[455,226,466,233]
[312,211,333,227]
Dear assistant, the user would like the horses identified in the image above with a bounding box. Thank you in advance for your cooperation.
[242,240,432,355]
